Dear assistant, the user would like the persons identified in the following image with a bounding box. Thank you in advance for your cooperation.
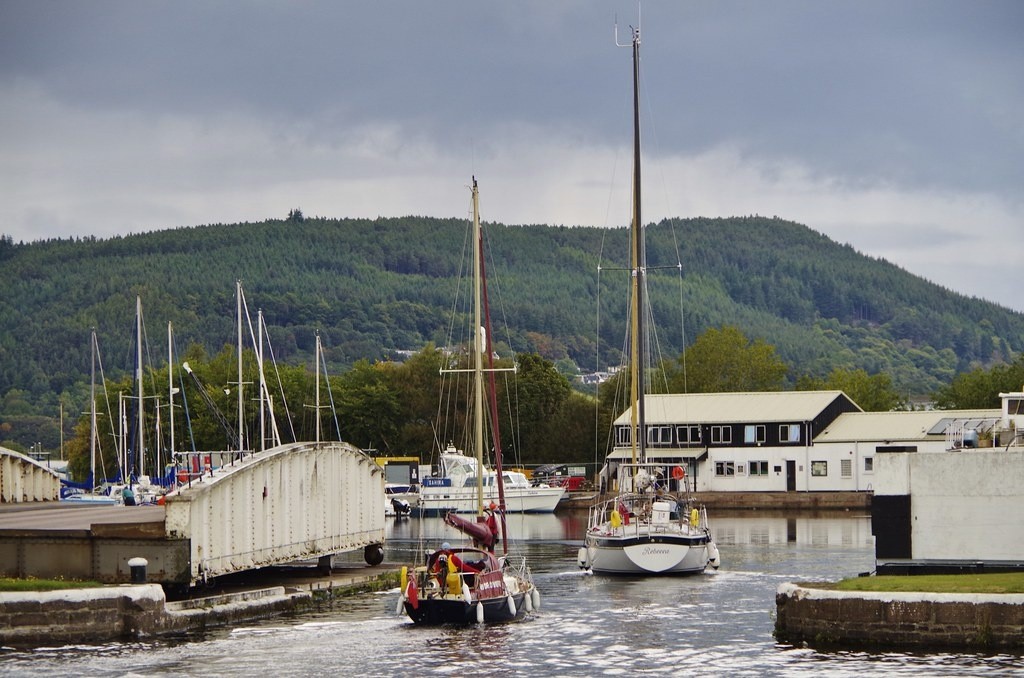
[122,486,136,506]
[434,542,480,587]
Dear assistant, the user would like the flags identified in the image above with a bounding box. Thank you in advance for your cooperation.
[408,574,418,609]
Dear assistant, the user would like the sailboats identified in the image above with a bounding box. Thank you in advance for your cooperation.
[386,176,567,513]
[401,226,540,628]
[577,0,720,578]
[58,280,342,503]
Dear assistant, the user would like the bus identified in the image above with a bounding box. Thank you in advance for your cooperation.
[531,463,603,491]
[508,464,541,479]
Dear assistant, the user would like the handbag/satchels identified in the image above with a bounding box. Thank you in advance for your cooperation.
[656,489,664,496]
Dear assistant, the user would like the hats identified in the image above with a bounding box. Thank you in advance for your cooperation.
[440,542,450,550]
[159,490,166,494]
[654,467,663,474]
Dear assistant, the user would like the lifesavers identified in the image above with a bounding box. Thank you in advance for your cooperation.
[672,465,686,481]
